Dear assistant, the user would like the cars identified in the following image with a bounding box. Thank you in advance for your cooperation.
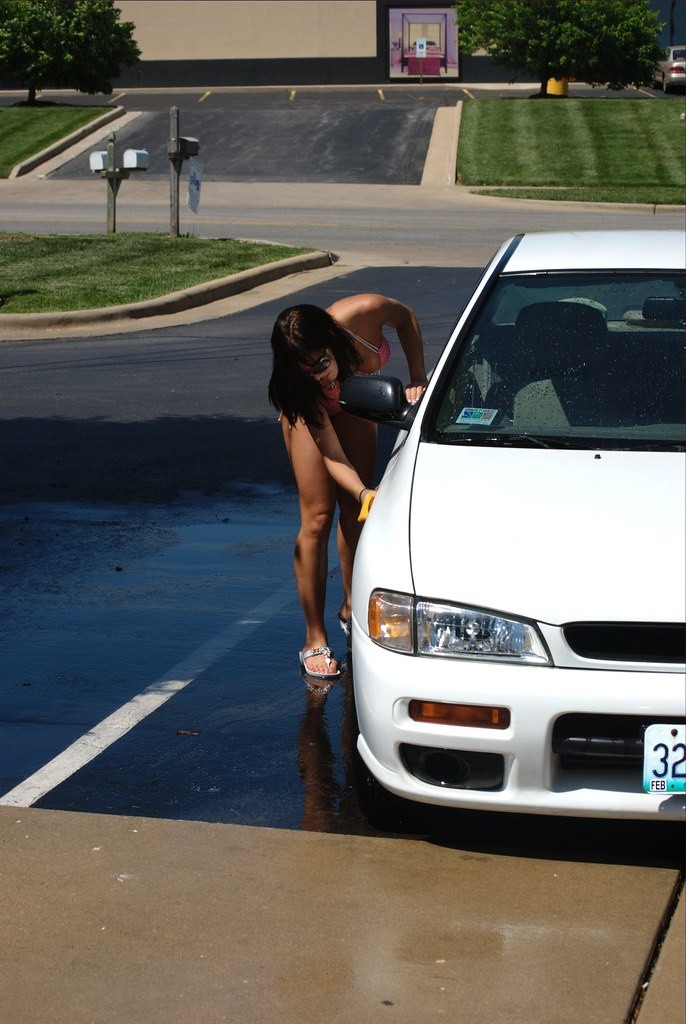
[336,228,686,822]
[651,45,686,93]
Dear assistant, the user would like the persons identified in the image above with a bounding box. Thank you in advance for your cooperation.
[268,293,428,678]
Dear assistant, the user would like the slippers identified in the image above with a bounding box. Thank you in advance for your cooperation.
[338,613,352,637]
[299,646,340,677]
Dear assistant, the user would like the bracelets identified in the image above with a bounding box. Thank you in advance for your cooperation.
[358,488,366,502]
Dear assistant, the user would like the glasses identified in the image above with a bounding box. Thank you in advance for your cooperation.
[306,347,333,377]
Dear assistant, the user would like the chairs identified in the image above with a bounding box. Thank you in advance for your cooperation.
[493,302,622,428]
